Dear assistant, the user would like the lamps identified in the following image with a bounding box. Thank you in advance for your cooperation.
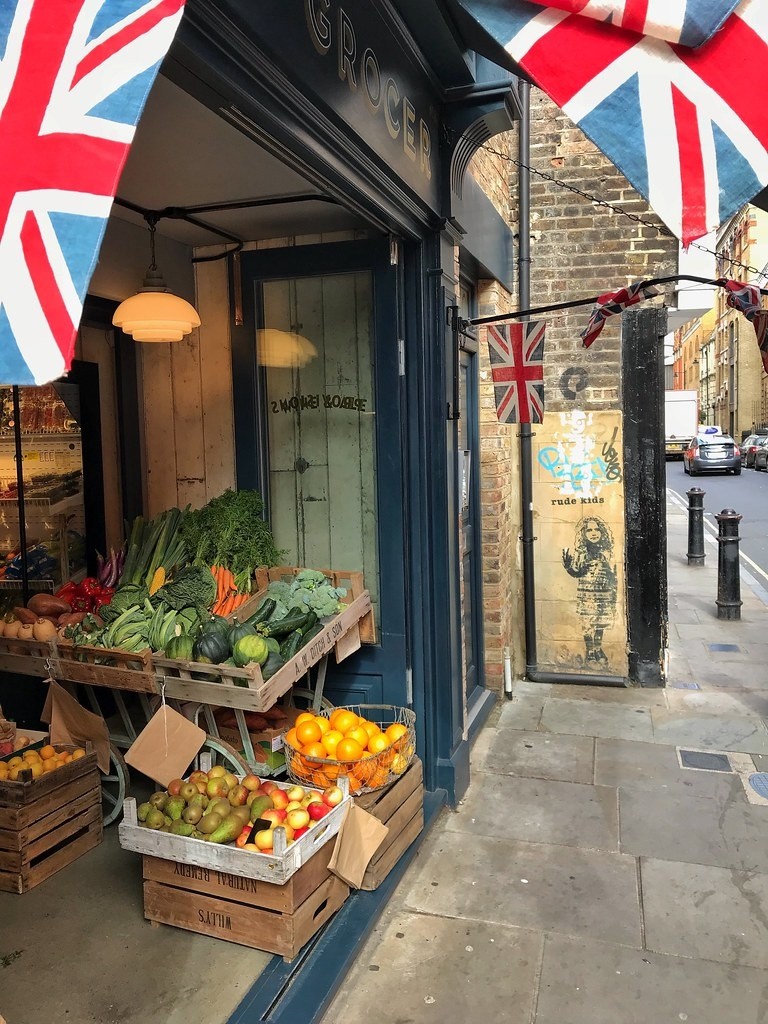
[112,215,202,343]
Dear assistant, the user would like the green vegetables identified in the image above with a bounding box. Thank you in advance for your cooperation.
[64,564,218,653]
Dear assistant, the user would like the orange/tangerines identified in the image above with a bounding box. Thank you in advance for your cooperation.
[284,708,415,795]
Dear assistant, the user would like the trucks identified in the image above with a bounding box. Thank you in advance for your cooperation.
[665,390,698,458]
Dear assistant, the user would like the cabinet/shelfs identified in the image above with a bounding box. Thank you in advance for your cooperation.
[0,430,88,596]
[150,567,369,714]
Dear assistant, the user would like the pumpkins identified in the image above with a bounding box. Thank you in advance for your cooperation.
[166,612,283,688]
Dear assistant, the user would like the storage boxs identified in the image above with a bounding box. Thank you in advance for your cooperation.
[144,833,350,964]
[197,704,308,777]
[0,636,64,680]
[57,565,268,694]
[0,728,104,894]
[284,754,424,891]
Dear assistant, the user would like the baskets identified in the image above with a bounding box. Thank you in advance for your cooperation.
[281,703,417,797]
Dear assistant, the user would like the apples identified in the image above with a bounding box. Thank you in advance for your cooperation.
[168,765,239,797]
[235,774,343,856]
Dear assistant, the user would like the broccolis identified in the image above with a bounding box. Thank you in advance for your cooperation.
[255,568,348,622]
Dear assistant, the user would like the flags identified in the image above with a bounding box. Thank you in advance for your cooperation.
[580,278,764,349]
[1,0,185,388]
[487,320,547,424]
[459,0,768,250]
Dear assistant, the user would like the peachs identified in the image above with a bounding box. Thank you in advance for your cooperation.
[0,745,86,782]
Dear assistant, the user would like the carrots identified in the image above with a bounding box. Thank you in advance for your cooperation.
[210,565,252,618]
[0,614,57,658]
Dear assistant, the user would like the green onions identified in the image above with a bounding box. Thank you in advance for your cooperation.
[120,502,194,587]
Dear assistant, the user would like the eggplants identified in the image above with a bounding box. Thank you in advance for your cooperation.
[93,539,126,587]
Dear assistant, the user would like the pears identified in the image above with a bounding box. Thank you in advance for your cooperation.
[138,783,274,845]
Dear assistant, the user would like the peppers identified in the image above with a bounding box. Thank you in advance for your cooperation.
[54,577,116,615]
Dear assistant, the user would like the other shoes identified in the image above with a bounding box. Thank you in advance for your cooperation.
[596,649,608,665]
[587,651,597,664]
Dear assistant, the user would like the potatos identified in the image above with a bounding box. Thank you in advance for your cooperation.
[11,593,104,628]
[215,707,294,734]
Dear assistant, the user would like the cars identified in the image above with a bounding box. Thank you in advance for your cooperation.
[682,435,743,476]
[754,435,768,471]
[739,434,767,468]
